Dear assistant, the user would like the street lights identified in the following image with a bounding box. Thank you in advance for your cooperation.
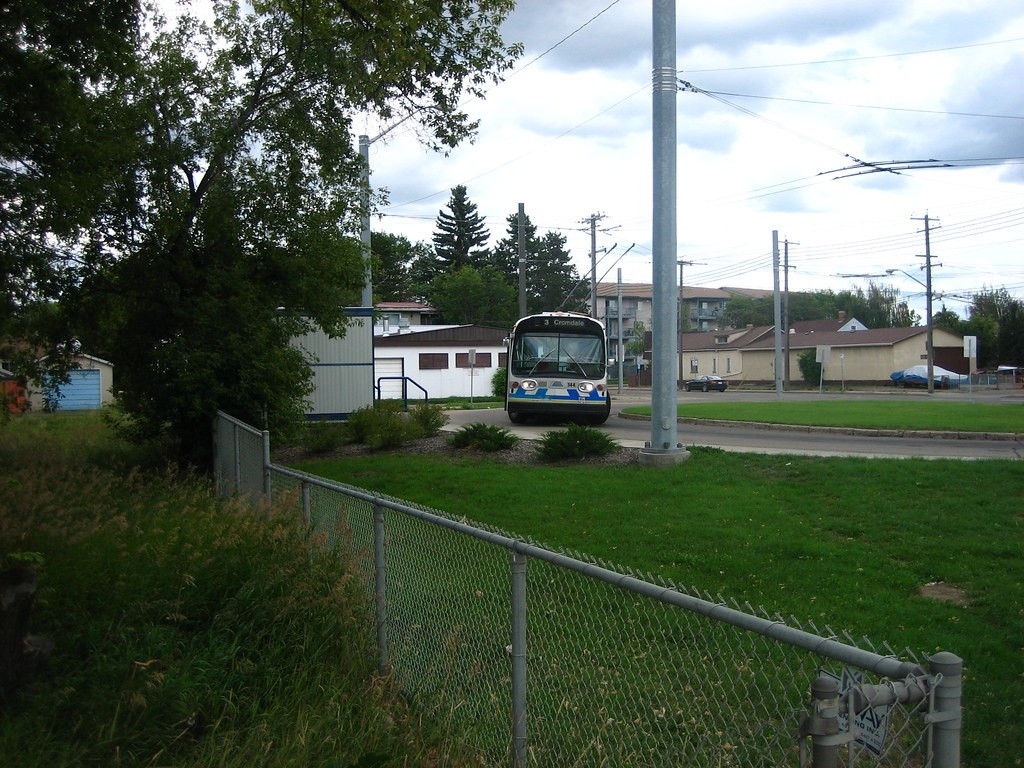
[884,268,935,392]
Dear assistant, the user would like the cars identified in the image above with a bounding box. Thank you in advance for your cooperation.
[684,375,728,393]
[890,365,969,390]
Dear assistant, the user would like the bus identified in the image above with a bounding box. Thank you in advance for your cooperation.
[502,309,615,428]
